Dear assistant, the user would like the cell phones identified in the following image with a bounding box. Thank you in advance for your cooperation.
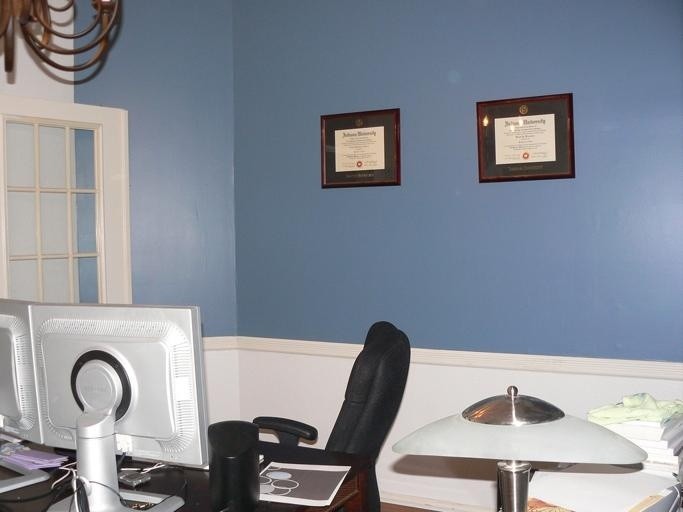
[117,469,152,486]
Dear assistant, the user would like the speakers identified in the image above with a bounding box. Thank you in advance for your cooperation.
[207,420,260,512]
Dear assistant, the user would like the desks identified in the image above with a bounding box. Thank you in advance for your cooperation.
[0,438,373,512]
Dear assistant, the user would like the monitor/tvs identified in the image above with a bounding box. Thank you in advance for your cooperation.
[0,296,51,494]
[30,301,208,512]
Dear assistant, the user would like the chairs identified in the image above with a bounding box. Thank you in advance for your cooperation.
[253,320,411,512]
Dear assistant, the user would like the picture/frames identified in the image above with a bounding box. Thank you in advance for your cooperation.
[321,108,402,189]
[476,93,576,183]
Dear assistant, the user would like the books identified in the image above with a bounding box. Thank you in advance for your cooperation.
[258,461,351,506]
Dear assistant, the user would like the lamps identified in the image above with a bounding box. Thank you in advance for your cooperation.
[391,385,648,512]
[0,0,120,73]
